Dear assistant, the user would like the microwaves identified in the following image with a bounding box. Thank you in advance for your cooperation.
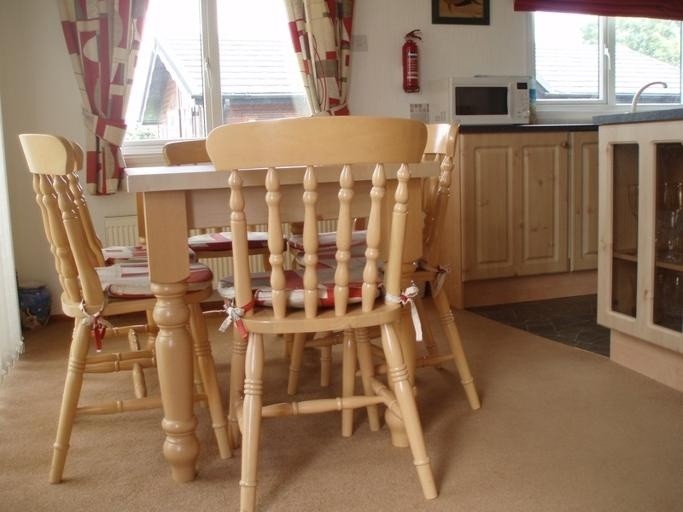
[429,75,531,127]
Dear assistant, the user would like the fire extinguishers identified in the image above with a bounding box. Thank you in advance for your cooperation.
[402,29,421,93]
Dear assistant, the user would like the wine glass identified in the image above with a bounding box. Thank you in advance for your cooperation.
[628,180,683,266]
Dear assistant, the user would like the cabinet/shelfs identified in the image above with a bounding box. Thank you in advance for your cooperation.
[595,119,683,355]
[459,131,598,282]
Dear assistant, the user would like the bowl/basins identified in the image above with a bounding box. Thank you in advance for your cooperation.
[19,288,52,331]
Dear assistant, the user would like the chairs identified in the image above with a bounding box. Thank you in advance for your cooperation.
[16,134,231,481]
[285,219,368,387]
[163,139,284,343]
[69,141,197,398]
[285,115,479,437]
[206,118,441,512]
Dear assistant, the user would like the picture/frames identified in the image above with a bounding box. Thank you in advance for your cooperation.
[431,1,490,26]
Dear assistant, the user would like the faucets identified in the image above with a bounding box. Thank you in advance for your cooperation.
[631,81,668,112]
